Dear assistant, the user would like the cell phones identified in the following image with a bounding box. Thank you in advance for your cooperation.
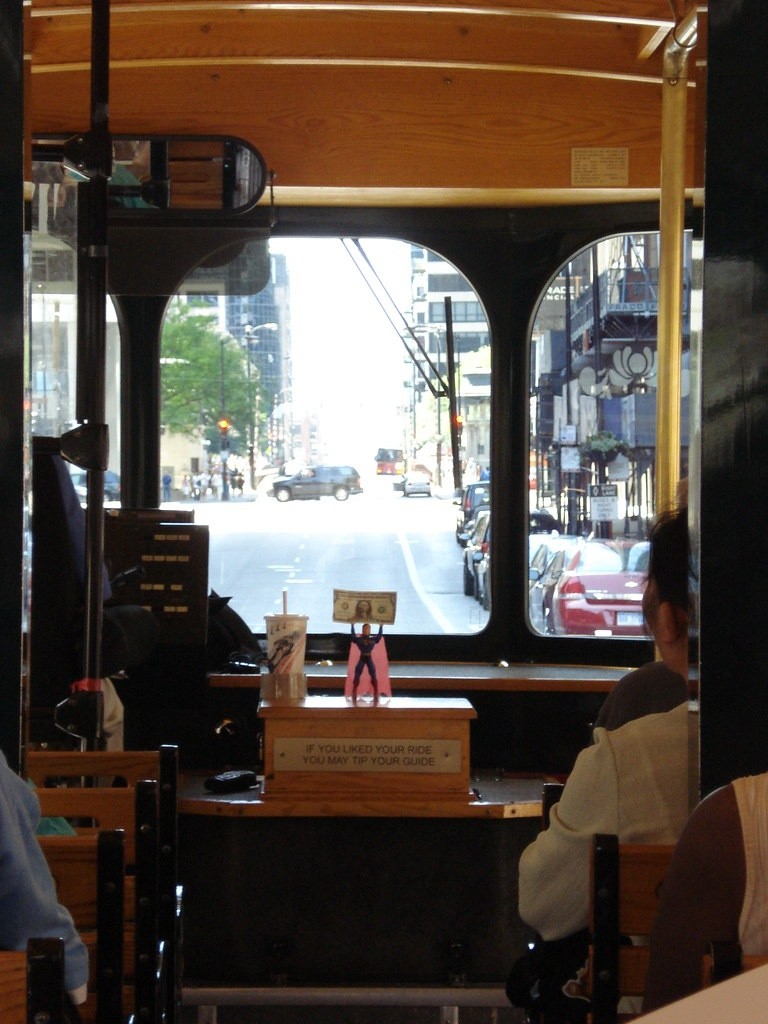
[204,769,263,793]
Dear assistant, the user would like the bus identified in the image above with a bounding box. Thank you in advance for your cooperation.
[374,447,405,475]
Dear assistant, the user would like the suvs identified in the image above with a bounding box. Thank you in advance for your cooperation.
[271,465,364,502]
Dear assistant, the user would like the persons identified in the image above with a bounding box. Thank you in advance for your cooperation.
[162,471,172,502]
[351,623,383,702]
[183,463,244,500]
[462,457,490,486]
[0,752,87,1005]
[518,501,768,1014]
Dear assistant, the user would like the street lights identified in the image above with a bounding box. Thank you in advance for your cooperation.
[246,321,280,488]
[219,334,262,501]
[412,322,459,490]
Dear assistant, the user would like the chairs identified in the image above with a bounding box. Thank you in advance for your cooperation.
[540,775,768,1024]
[0,741,180,1024]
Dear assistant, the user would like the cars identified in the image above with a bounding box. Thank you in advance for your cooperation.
[393,471,432,498]
[452,481,651,636]
[69,470,120,504]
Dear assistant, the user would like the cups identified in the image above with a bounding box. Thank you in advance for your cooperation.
[264,614,310,674]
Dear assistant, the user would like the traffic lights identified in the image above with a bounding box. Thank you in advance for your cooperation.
[219,420,229,440]
